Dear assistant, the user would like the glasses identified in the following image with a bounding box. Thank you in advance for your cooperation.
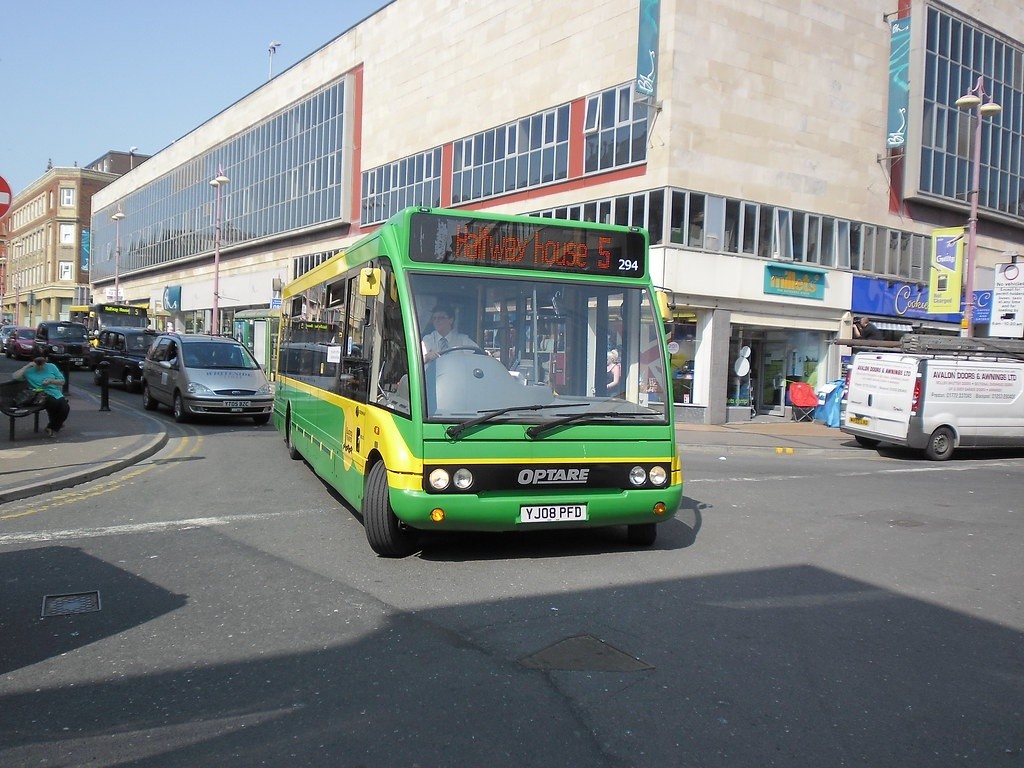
[433,316,448,321]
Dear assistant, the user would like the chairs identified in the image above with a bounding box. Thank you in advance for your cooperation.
[791,382,819,421]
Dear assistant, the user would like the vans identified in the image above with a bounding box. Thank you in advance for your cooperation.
[839,349,1024,462]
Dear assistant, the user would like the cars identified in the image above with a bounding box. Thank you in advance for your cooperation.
[5,327,38,361]
[0,325,24,353]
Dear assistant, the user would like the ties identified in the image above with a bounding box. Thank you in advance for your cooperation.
[441,339,448,356]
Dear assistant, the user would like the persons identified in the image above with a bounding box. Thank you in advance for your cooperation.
[12,349,70,439]
[420,305,494,372]
[853,316,884,340]
[607,349,620,397]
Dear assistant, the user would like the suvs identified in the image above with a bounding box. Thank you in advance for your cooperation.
[34,320,95,369]
[89,325,167,394]
[142,332,273,425]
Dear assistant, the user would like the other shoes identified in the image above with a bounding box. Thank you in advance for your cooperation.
[46,427,56,438]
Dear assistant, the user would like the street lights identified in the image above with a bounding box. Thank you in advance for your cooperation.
[955,75,1003,338]
[209,163,232,337]
[14,237,24,326]
[111,203,126,304]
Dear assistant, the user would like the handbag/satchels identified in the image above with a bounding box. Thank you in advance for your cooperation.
[17,387,46,407]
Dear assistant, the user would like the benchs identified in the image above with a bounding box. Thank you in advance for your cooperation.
[0,379,48,440]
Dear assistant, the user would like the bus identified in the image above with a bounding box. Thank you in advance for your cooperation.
[272,205,684,558]
[69,303,153,348]
